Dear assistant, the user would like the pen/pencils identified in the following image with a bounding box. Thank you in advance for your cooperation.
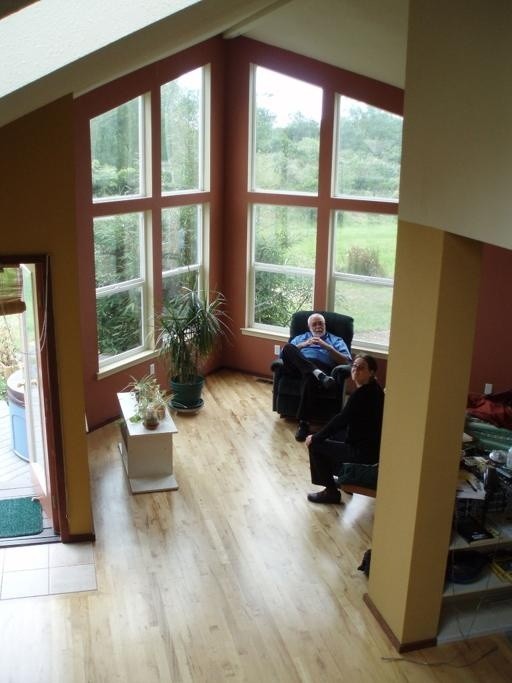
[466,479,477,491]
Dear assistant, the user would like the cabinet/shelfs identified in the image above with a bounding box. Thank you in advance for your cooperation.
[114,392,182,496]
[436,508,512,647]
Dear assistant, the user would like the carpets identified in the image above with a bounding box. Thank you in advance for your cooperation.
[0,494,43,539]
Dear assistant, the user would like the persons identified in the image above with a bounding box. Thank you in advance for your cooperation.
[280,312,353,441]
[306,350,385,504]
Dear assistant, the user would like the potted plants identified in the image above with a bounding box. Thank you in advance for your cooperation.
[157,289,233,415]
[131,377,165,430]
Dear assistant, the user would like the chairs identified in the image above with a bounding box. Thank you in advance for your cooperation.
[271,309,355,423]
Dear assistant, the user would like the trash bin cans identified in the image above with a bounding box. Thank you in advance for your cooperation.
[6,372,29,462]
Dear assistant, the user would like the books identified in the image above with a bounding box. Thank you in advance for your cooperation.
[455,516,497,546]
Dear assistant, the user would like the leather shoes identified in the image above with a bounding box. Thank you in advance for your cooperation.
[317,372,337,388]
[295,419,311,443]
[307,489,342,503]
[333,476,354,494]
